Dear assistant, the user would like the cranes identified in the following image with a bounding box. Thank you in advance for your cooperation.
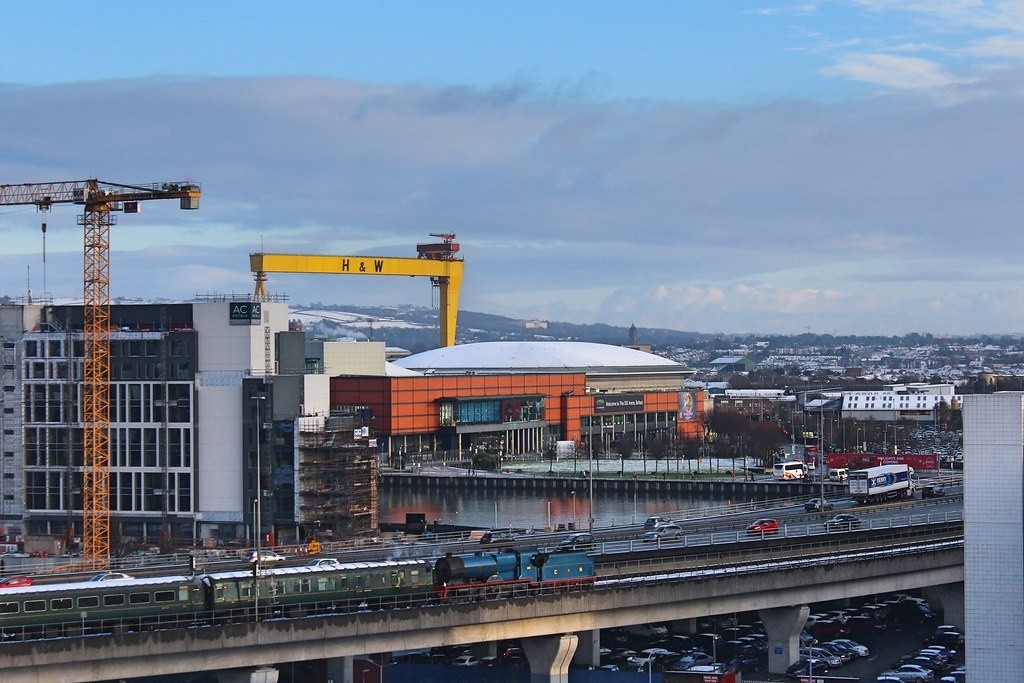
[1,178,202,571]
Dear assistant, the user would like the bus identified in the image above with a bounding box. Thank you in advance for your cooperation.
[773,462,805,480]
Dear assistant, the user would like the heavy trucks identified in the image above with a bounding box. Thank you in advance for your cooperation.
[803,445,819,466]
[848,464,917,504]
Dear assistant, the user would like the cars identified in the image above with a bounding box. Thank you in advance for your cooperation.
[306,558,340,566]
[805,498,834,512]
[395,647,524,667]
[643,517,684,543]
[599,594,964,683]
[922,485,947,499]
[0,575,32,588]
[242,550,286,563]
[557,533,596,551]
[824,514,861,530]
[479,532,515,545]
[746,519,779,536]
[89,573,135,582]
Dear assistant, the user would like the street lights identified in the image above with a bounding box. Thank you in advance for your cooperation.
[251,396,266,564]
[581,387,597,533]
[254,499,258,550]
[648,649,668,683]
[810,639,818,683]
[366,658,397,683]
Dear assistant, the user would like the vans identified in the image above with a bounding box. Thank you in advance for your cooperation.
[830,469,848,482]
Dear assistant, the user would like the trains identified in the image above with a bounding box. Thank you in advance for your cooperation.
[1,547,597,642]
